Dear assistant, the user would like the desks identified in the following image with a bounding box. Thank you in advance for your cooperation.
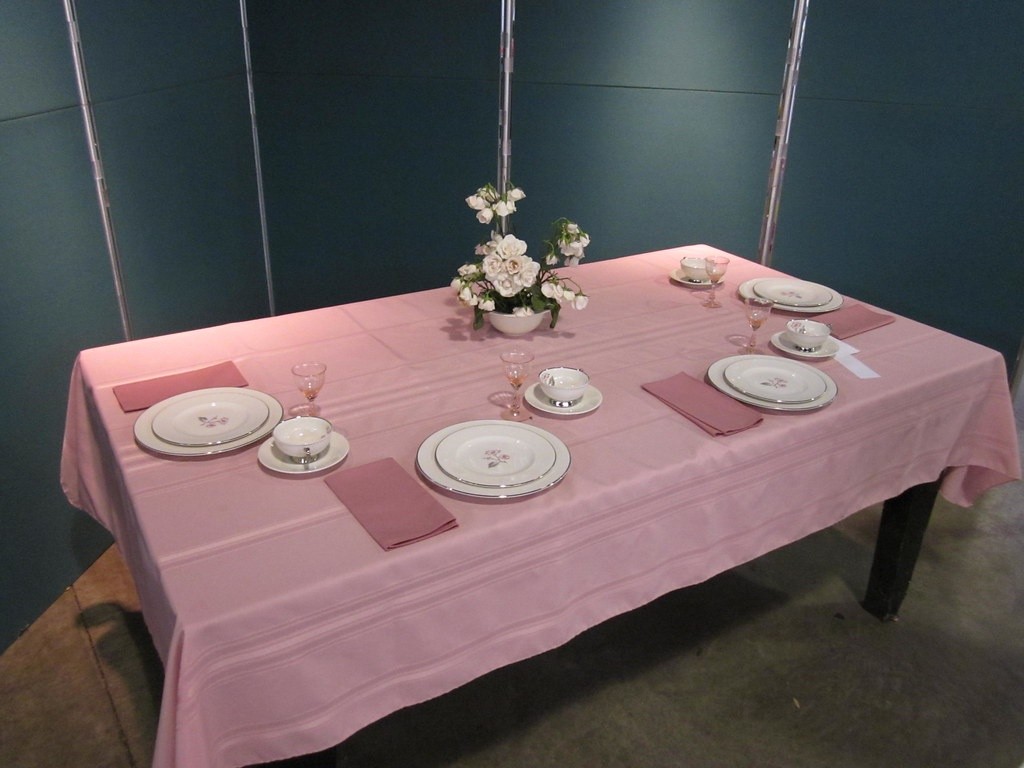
[60,243,1023,768]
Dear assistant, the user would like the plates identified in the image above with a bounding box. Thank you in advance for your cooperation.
[736,276,845,313]
[133,386,284,457]
[669,267,726,290]
[770,330,841,360]
[524,382,603,415]
[416,419,570,500]
[257,431,350,474]
[706,354,838,412]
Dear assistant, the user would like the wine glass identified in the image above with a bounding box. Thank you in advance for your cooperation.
[738,297,775,354]
[499,348,536,422]
[292,361,327,416]
[700,255,730,308]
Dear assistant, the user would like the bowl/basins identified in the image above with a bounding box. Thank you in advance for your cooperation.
[785,318,833,352]
[538,367,591,407]
[272,416,333,464]
[680,257,712,283]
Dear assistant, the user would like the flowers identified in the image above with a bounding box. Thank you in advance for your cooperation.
[451,180,592,330]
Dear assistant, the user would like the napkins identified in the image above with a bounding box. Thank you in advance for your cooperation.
[808,305,896,339]
[641,371,763,437]
[111,360,249,412]
[324,458,459,552]
